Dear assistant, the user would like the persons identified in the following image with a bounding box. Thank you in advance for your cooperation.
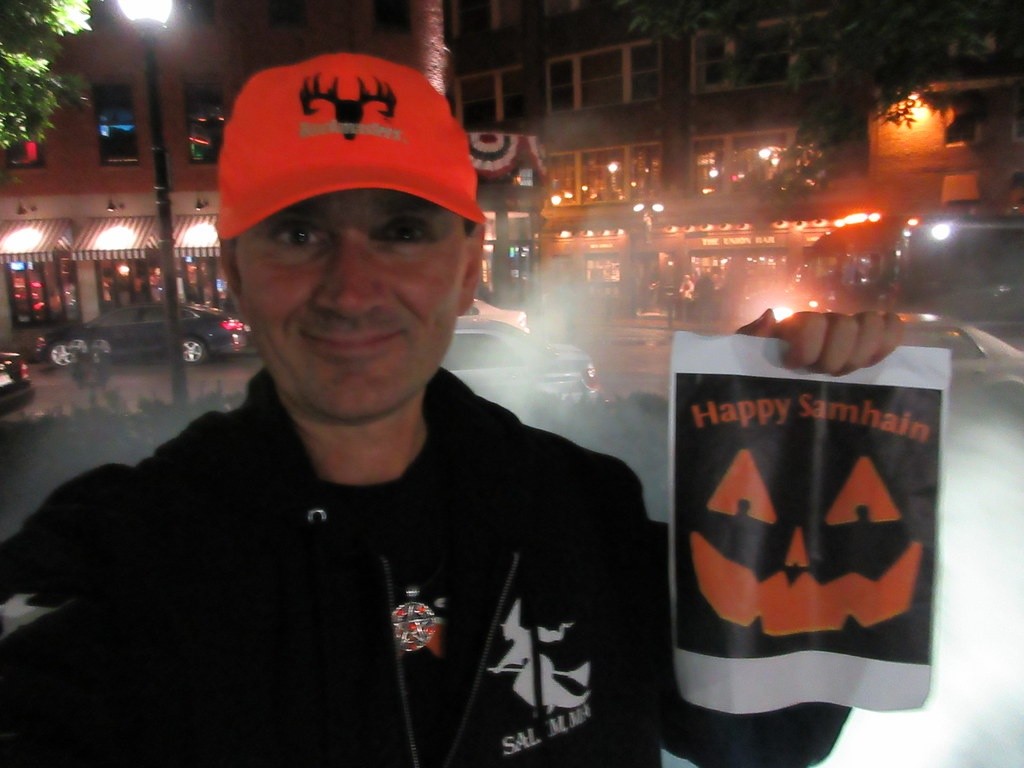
[0,56,901,767]
[679,275,694,301]
[70,348,129,413]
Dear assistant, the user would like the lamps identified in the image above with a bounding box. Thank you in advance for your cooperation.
[194,193,209,211]
[15,193,37,216]
[105,193,125,214]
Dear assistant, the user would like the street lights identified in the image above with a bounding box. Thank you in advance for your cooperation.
[632,198,665,317]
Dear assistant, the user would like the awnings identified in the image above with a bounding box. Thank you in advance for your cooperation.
[0,218,73,262]
[173,214,221,257]
[71,218,160,261]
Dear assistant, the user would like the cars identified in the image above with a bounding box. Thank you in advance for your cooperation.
[42,301,253,367]
[457,299,531,333]
[0,351,36,419]
[878,309,1024,446]
[435,320,601,415]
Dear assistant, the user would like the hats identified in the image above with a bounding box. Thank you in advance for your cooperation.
[215,53,488,240]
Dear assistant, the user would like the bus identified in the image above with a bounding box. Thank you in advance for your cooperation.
[799,211,1024,322]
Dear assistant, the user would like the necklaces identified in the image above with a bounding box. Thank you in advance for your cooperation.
[392,558,446,652]
